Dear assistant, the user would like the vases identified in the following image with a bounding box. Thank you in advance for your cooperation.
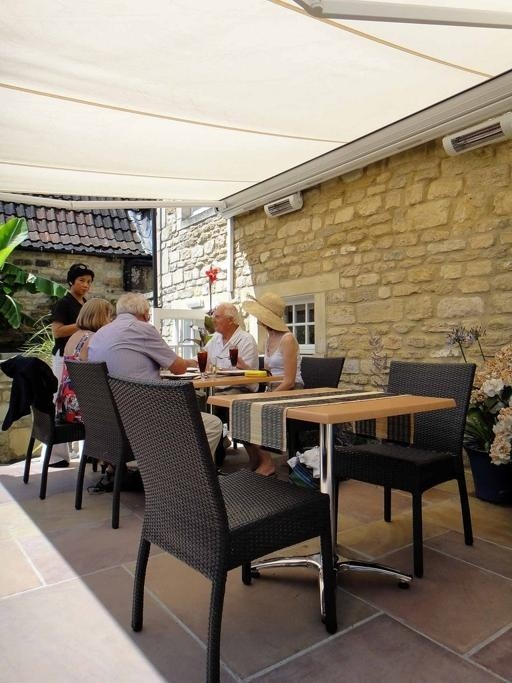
[465,449,512,508]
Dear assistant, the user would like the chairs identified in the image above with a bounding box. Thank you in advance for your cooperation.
[351,360,473,579]
[228,356,264,450]
[64,360,136,529]
[262,356,346,475]
[107,373,338,683]
[23,393,85,500]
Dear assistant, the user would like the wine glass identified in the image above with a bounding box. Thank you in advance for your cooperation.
[196,347,209,380]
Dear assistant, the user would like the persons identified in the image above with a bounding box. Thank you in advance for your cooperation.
[43,260,95,467]
[63,300,135,481]
[89,293,222,490]
[227,293,304,477]
[182,302,260,448]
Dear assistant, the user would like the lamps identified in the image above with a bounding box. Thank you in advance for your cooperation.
[264,190,304,218]
[442,111,512,157]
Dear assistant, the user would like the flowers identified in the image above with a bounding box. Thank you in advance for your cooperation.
[445,324,512,465]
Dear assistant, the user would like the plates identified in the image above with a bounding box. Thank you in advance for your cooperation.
[212,368,256,375]
[161,367,199,380]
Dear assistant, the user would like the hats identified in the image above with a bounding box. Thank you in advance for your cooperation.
[243,293,290,331]
[67,263,94,281]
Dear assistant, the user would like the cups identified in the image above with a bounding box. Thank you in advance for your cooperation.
[228,344,241,370]
